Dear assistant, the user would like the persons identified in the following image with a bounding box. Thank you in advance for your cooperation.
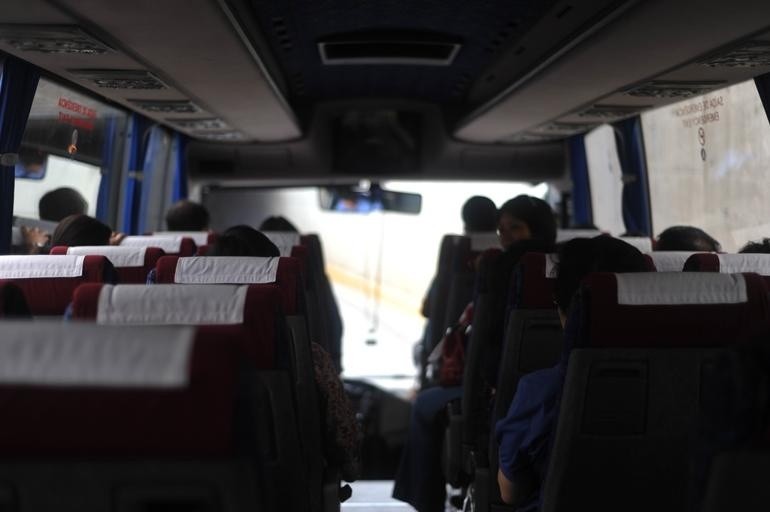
[20,186,362,511]
[391,193,770,512]
[334,195,369,211]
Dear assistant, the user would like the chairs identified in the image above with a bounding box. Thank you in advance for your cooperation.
[64,281,342,512]
[0,317,271,510]
[428,226,770,481]
[0,225,344,455]
[531,268,770,511]
[467,251,660,512]
